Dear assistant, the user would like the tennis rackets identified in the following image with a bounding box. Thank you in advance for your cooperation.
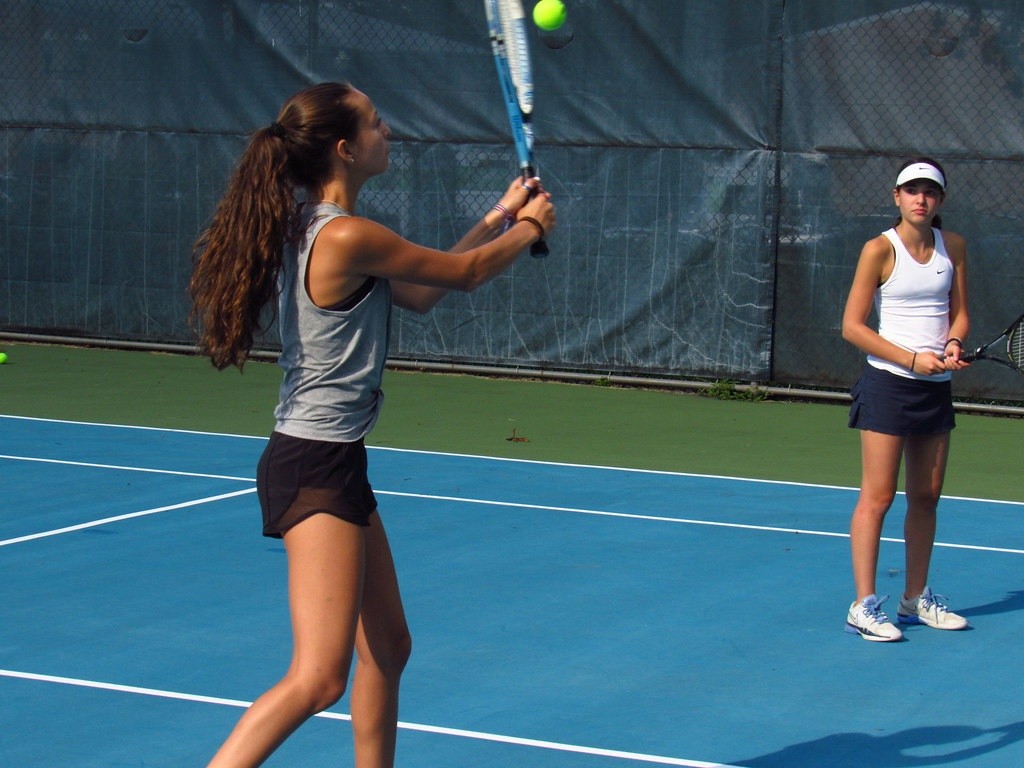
[936,313,1024,374]
[485,0,547,258]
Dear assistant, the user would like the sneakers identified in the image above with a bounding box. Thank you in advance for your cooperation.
[844,594,903,642]
[897,586,969,630]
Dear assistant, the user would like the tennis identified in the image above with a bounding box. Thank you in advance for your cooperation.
[533,0,567,30]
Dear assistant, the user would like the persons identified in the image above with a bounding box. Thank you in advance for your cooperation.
[183,81,557,768]
[841,156,971,643]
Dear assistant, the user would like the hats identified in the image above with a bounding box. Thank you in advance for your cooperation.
[897,162,945,191]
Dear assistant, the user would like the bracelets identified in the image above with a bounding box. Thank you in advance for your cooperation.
[911,353,916,372]
[945,336,963,349]
[494,202,513,230]
[517,216,545,238]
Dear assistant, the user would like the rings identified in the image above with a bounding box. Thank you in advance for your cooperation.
[523,184,532,192]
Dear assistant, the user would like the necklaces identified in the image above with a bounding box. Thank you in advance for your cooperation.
[306,200,350,214]
